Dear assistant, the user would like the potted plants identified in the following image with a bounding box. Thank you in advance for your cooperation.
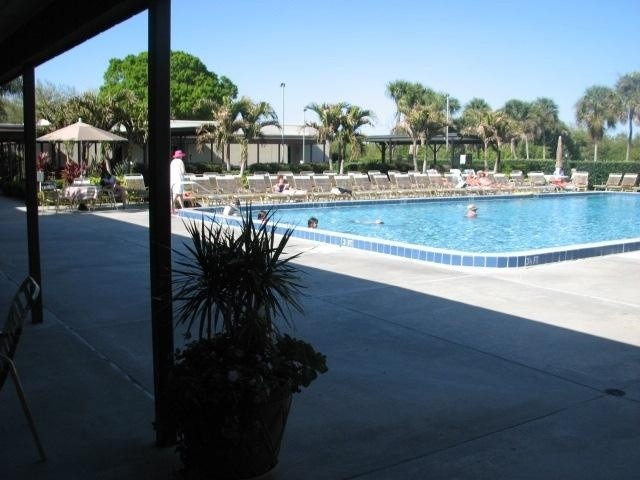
[166,200,329,477]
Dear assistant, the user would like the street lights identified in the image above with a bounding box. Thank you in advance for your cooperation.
[303,108,308,163]
[281,81,286,165]
[446,92,452,161]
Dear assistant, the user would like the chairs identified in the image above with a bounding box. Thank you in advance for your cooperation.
[1,275,48,466]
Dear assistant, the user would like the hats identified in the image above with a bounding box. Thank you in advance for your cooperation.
[172,150,186,159]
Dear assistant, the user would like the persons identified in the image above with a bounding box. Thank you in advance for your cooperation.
[477,172,513,188]
[566,167,577,187]
[259,211,267,221]
[170,151,185,214]
[272,175,306,195]
[464,204,477,219]
[465,171,480,187]
[549,165,568,187]
[101,169,130,207]
[307,216,318,229]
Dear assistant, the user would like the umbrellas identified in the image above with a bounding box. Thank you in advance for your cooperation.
[35,118,130,173]
